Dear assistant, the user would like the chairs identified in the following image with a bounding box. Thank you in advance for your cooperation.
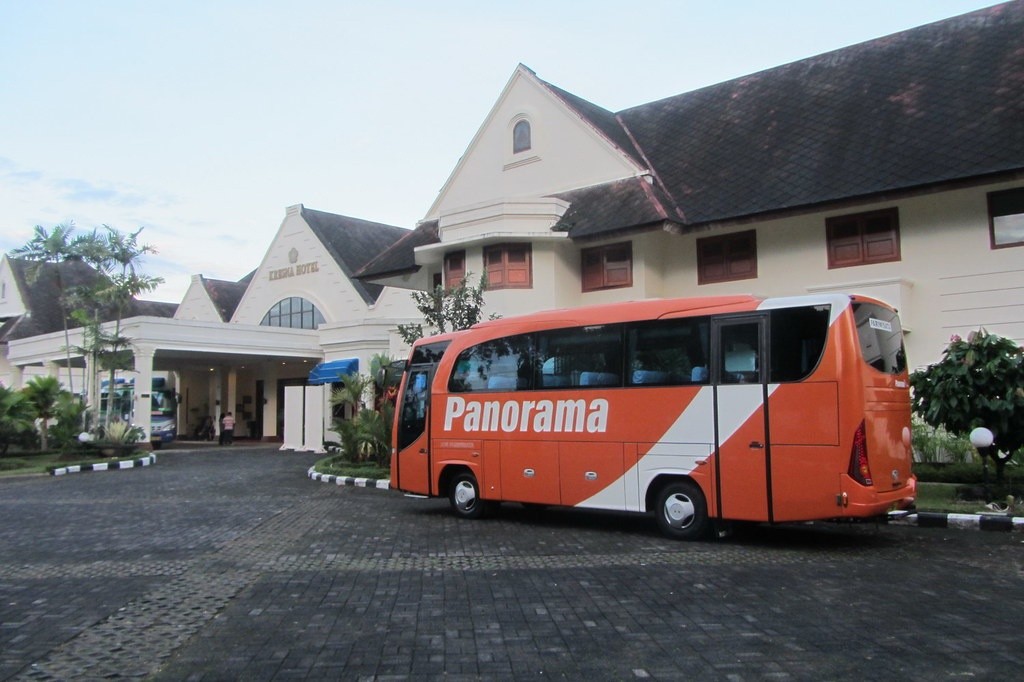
[488,376,528,390]
[543,375,569,386]
[579,372,620,386]
[691,367,709,383]
[631,370,669,385]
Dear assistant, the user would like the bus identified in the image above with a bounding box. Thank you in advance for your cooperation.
[375,290,918,542]
[100,377,184,450]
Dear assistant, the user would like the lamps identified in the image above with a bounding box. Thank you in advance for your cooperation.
[969,427,994,505]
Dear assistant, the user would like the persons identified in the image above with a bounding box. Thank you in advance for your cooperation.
[218,412,235,444]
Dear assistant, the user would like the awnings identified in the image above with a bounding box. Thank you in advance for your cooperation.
[308,358,359,386]
[97,377,164,389]
